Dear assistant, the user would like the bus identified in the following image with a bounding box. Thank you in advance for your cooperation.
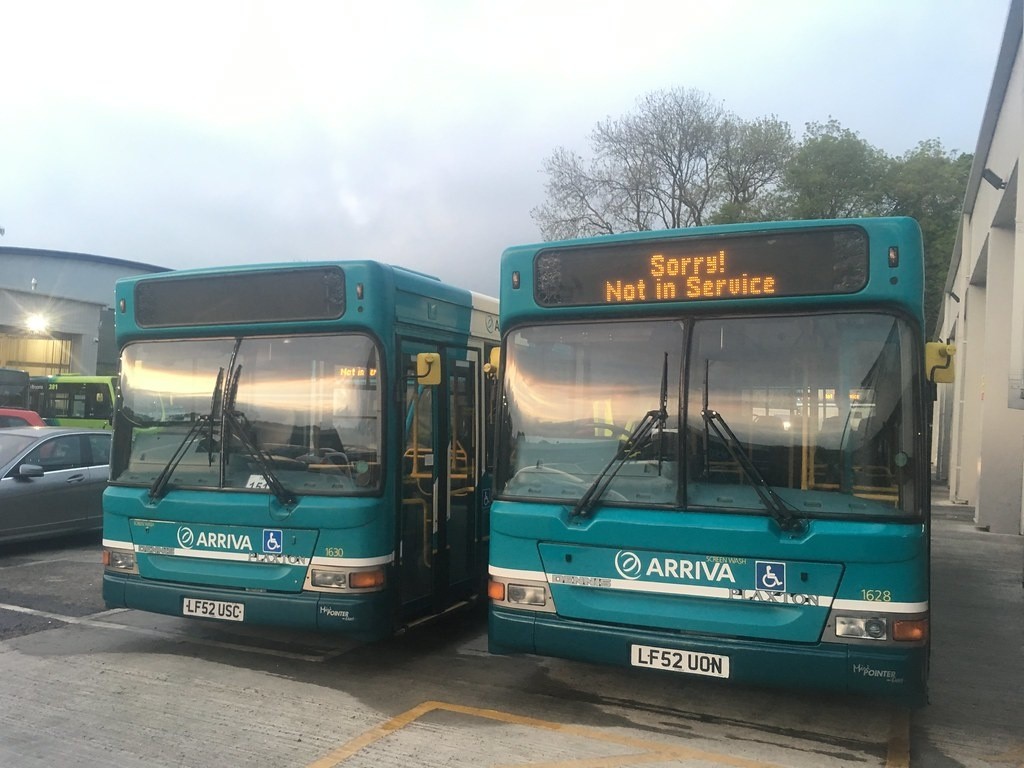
[0,368,31,430]
[488,222,962,724]
[104,259,539,673]
[28,371,175,450]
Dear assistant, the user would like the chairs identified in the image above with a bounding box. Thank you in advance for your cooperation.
[619,412,903,504]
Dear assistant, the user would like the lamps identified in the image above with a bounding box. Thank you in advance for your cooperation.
[980,168,1008,189]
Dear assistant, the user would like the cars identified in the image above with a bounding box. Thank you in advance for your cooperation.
[0,408,49,464]
[0,425,132,553]
